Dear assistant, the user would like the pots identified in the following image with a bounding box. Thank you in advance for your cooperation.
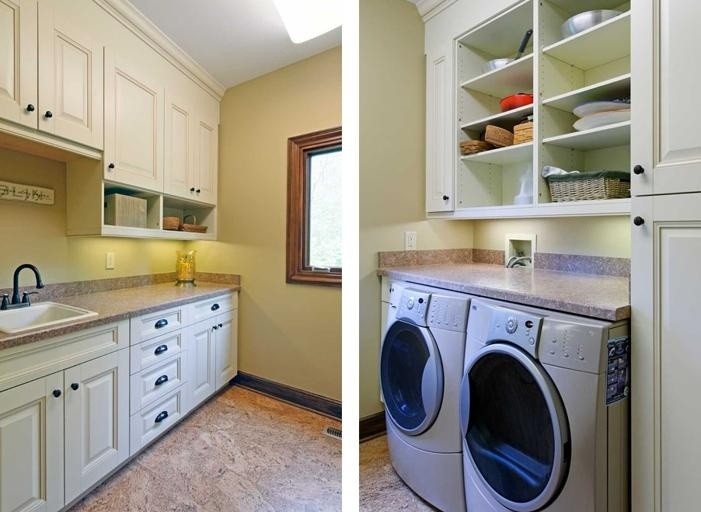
[486,27,532,71]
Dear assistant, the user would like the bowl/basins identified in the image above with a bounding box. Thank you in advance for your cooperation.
[561,9,627,39]
[497,92,534,111]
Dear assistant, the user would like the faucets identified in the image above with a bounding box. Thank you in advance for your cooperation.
[11,263,45,304]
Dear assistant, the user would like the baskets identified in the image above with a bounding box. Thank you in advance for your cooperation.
[460,122,533,155]
[164,216,181,229]
[183,214,207,234]
[547,169,631,201]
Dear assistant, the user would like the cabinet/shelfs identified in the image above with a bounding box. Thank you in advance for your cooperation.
[188,288,239,411]
[129,305,188,449]
[2,318,129,512]
[168,53,226,245]
[2,0,106,160]
[63,2,169,239]
[415,0,634,217]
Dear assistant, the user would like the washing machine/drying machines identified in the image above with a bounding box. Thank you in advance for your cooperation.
[457,296,632,512]
[379,279,476,512]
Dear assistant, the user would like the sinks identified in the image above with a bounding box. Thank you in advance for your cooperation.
[0,301,99,334]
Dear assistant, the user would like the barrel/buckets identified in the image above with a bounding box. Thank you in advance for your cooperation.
[512,155,533,205]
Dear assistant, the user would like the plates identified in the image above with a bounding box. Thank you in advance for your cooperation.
[572,109,631,132]
[572,100,631,118]
